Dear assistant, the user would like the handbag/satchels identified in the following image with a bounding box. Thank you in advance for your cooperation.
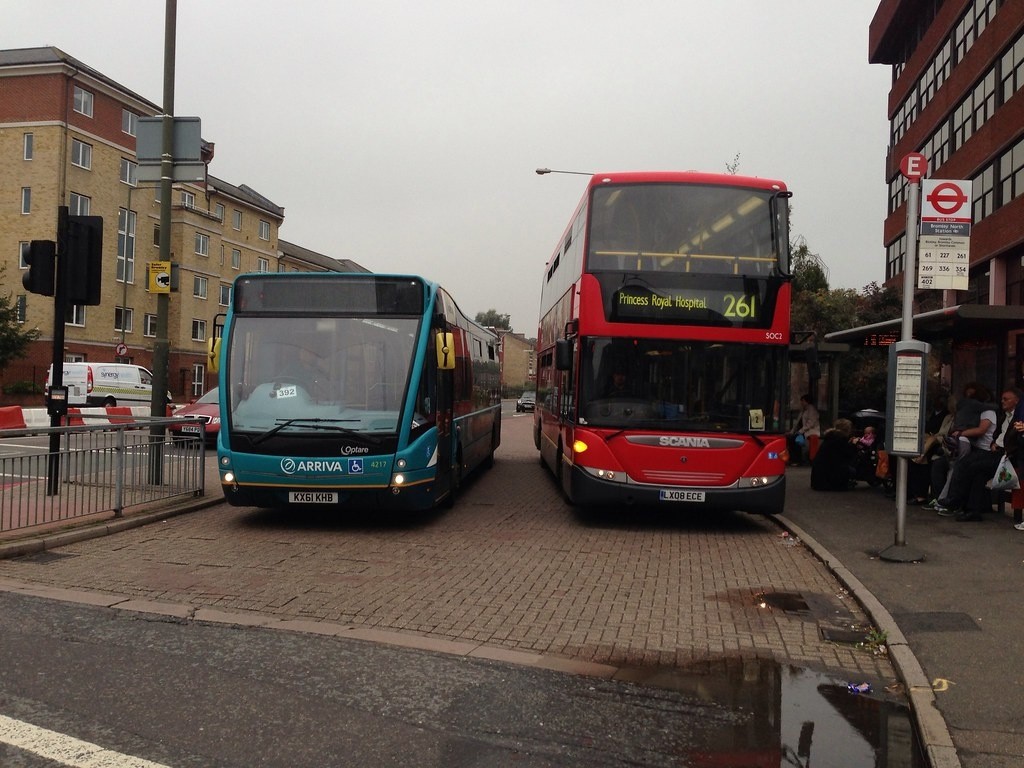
[795,434,805,446]
[991,455,1021,491]
[875,449,891,478]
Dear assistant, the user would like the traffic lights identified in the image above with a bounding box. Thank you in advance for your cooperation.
[22,239,56,295]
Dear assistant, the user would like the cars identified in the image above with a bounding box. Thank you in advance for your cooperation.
[515,391,536,413]
[167,384,224,447]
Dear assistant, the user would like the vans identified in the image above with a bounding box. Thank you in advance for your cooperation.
[44,362,171,417]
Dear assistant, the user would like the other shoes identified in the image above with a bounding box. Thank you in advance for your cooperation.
[921,498,945,510]
[1014,521,1024,531]
[790,462,802,466]
[955,510,983,522]
[907,495,929,505]
[912,455,927,465]
[938,507,964,516]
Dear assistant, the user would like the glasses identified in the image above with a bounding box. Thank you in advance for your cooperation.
[1002,396,1016,402]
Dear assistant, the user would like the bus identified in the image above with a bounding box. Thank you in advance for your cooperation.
[532,171,822,521]
[206,272,503,517]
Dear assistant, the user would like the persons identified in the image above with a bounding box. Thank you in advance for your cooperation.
[607,367,627,399]
[274,341,328,403]
[906,382,1024,530]
[810,418,859,492]
[857,427,876,450]
[785,394,821,465]
